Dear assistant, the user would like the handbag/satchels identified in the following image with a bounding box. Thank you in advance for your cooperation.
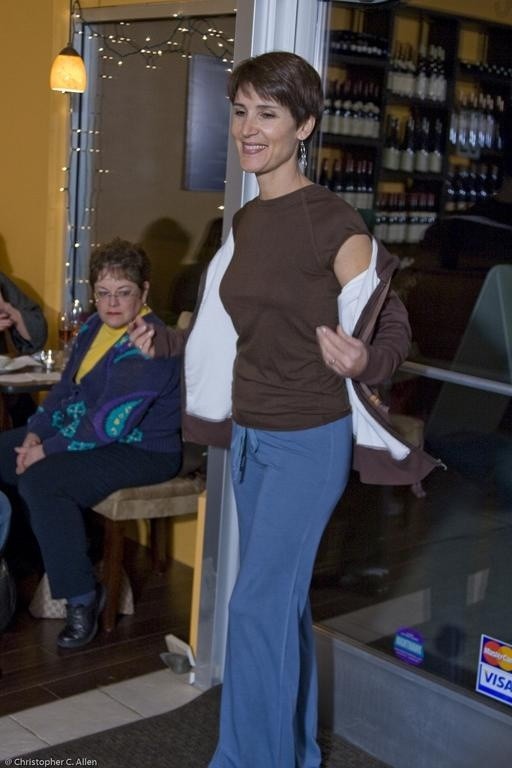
[28,563,135,618]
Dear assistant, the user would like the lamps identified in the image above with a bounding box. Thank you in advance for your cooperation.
[48,1,88,94]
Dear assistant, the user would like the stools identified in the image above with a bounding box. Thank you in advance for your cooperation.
[90,475,198,634]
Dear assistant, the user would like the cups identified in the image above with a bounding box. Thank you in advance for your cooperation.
[40,349,54,373]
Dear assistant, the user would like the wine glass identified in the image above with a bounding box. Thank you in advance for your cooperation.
[57,302,84,351]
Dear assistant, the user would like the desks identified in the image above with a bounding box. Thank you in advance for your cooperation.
[0,354,61,394]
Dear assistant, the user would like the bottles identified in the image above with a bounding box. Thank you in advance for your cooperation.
[319,30,504,245]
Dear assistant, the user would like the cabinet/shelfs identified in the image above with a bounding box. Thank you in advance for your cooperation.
[312,1,511,254]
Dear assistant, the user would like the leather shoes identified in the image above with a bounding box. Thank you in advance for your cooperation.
[56,582,107,650]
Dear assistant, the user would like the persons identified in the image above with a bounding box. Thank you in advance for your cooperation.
[0,235,184,648]
[424,182,511,271]
[127,53,417,768]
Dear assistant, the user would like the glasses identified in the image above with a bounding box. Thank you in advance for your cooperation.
[93,290,135,299]
